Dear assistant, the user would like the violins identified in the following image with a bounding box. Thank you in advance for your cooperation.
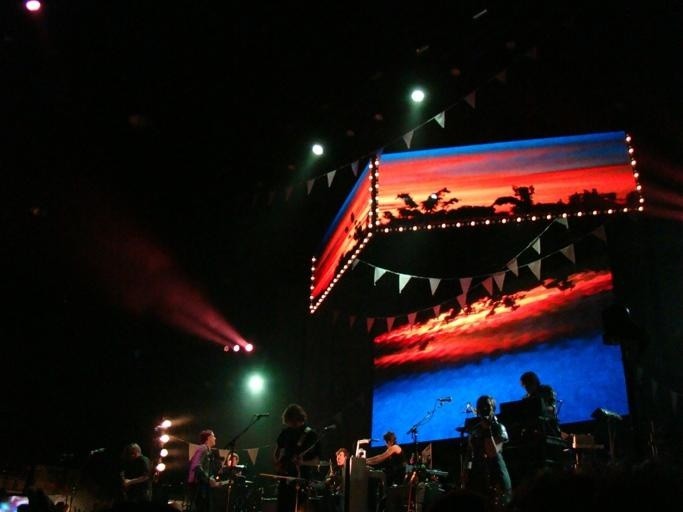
[483,416,500,426]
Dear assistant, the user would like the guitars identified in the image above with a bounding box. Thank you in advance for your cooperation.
[120,472,128,501]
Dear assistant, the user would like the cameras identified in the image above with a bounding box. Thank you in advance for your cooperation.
[0,492,30,512]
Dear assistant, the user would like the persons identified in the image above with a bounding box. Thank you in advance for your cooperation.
[119,442,154,512]
[467,395,514,512]
[215,451,243,481]
[187,428,223,512]
[366,432,407,487]
[332,447,350,475]
[273,403,323,511]
[514,371,564,443]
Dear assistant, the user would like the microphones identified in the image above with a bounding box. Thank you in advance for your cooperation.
[371,438,380,442]
[89,447,107,456]
[321,424,337,433]
[436,396,453,402]
[253,412,270,417]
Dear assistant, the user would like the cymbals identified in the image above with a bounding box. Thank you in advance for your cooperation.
[301,460,333,467]
